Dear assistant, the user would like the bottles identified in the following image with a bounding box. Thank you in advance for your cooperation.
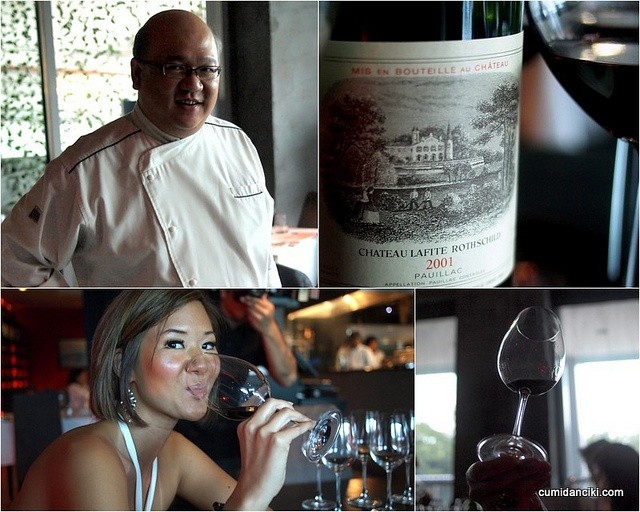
[319,0,523,288]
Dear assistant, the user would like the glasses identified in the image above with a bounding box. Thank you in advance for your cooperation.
[137,57,222,81]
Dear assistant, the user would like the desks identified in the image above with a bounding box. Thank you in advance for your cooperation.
[272,227,318,288]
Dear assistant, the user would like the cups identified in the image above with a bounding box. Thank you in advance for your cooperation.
[274,213,287,233]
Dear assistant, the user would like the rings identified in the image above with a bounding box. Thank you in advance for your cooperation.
[493,491,518,510]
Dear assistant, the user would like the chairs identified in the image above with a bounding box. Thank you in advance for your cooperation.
[13,389,62,494]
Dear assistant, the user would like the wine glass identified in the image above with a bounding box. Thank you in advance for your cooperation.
[187,351,341,465]
[367,412,410,510]
[321,416,358,511]
[391,412,413,506]
[524,2,639,287]
[474,304,567,465]
[301,421,338,510]
[342,411,383,508]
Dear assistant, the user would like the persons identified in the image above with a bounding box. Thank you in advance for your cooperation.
[68,367,90,409]
[334,331,384,372]
[8,290,317,510]
[2,10,282,288]
[407,186,434,210]
[464,458,554,510]
[175,292,301,480]
